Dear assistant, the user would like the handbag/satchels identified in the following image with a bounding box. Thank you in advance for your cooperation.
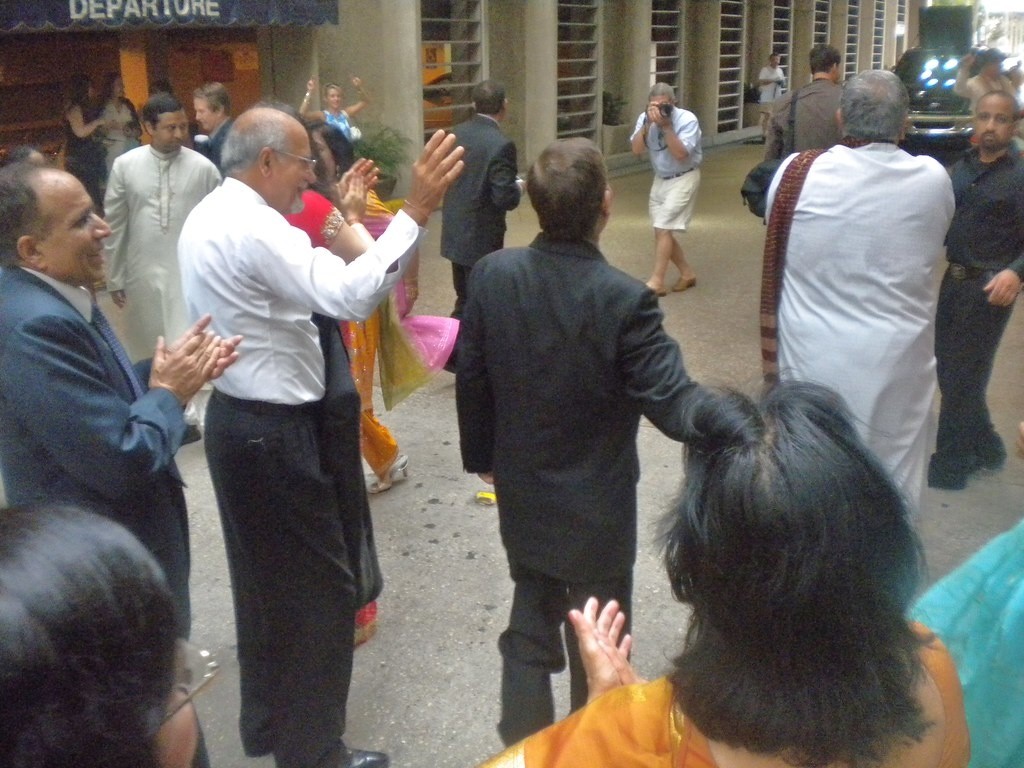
[742,160,784,224]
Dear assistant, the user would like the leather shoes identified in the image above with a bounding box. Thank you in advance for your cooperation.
[329,748,389,768]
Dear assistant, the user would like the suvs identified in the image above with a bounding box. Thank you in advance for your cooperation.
[888,45,1024,159]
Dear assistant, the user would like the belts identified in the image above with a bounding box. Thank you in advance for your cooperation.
[946,263,999,281]
[662,166,694,180]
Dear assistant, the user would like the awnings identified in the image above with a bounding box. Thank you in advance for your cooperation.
[0,0,339,31]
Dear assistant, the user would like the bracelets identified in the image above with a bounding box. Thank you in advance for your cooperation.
[304,94,311,103]
[404,200,429,218]
[348,219,361,226]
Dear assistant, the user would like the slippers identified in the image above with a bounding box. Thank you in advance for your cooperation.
[654,288,666,297]
[671,276,696,291]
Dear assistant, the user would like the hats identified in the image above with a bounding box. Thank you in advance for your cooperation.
[978,48,1006,69]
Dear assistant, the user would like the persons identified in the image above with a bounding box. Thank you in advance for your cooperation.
[765,69,957,511]
[0,502,217,768]
[957,48,1024,117]
[176,107,464,768]
[758,53,786,131]
[63,70,143,203]
[456,137,707,747]
[629,82,702,293]
[766,43,846,160]
[0,163,243,642]
[300,76,370,139]
[100,91,223,367]
[927,89,1024,489]
[484,379,970,768]
[194,80,234,165]
[440,79,527,374]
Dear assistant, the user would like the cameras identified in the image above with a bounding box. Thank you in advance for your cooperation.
[654,101,675,117]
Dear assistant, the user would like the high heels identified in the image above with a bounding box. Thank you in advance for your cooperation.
[368,454,408,495]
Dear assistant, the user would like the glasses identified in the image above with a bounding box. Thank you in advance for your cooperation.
[162,637,219,724]
[267,143,317,174]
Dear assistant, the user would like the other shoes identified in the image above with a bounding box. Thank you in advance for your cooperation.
[180,426,201,448]
[353,600,378,643]
[927,441,1006,489]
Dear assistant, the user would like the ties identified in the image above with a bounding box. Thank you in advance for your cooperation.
[90,302,187,487]
